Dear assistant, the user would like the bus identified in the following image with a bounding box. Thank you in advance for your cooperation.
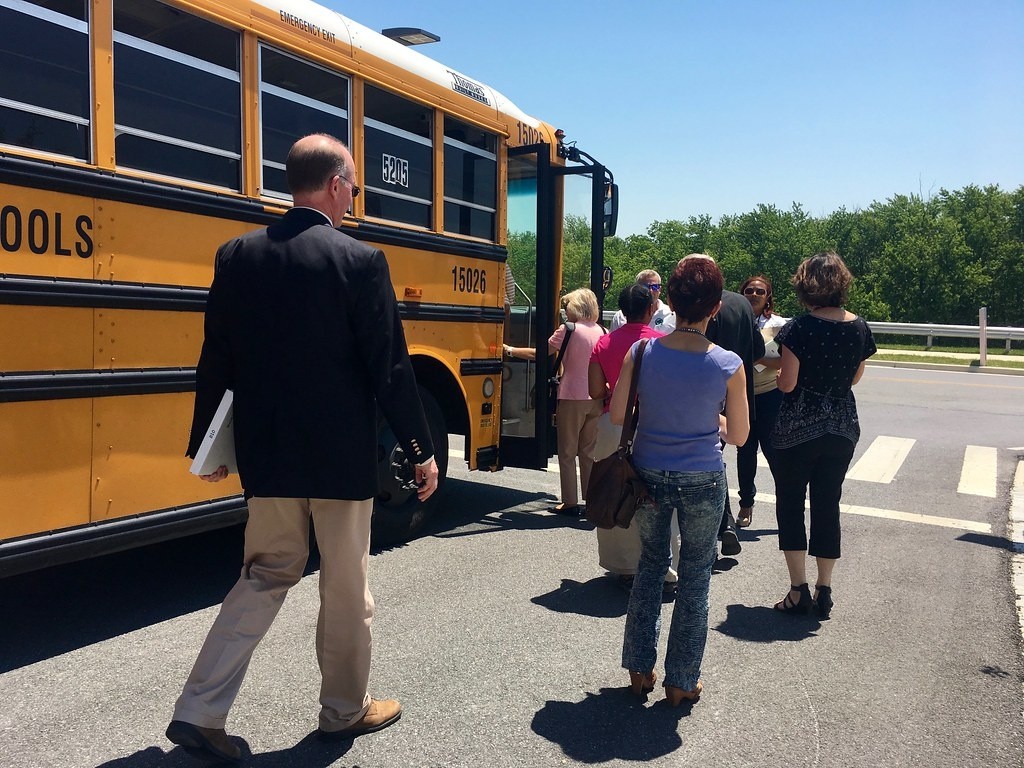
[0,0,619,582]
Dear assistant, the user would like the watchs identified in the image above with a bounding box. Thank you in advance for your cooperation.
[507,347,514,358]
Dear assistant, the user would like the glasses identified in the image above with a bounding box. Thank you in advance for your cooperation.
[332,174,362,197]
[642,282,662,291]
[743,287,768,296]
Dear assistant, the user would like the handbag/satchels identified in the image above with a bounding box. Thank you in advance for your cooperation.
[546,377,560,404]
[585,450,647,528]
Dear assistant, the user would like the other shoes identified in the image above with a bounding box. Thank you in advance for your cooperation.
[549,504,580,519]
[737,504,753,526]
[722,529,741,556]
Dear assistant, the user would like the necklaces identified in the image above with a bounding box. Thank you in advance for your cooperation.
[674,328,706,337]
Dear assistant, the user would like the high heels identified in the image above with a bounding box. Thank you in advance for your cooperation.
[813,583,834,619]
[631,666,656,695]
[774,583,814,615]
[665,680,703,707]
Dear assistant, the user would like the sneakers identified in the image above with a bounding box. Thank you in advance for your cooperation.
[166,720,242,763]
[319,701,402,737]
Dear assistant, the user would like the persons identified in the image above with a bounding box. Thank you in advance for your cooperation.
[775,253,877,612]
[611,268,677,336]
[677,252,765,557]
[165,131,440,764]
[607,254,751,709]
[502,289,612,516]
[736,275,788,531]
[586,285,680,597]
